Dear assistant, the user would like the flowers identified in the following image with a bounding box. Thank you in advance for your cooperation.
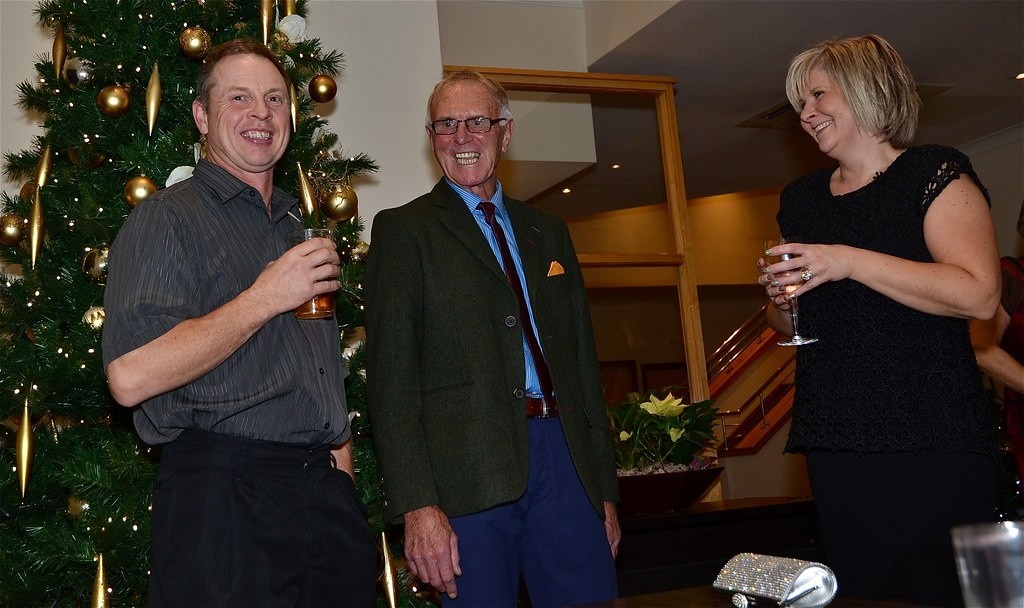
[606,385,721,475]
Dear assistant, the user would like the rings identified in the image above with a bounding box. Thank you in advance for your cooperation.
[801,267,813,281]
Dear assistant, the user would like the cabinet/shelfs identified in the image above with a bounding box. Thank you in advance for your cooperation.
[617,497,824,600]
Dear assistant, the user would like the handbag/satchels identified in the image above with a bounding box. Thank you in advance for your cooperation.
[713,553,838,608]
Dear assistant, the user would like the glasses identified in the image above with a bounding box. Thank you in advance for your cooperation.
[430,116,507,135]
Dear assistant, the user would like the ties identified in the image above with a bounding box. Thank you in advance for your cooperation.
[475,201,553,404]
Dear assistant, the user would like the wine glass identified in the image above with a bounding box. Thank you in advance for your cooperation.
[763,239,819,345]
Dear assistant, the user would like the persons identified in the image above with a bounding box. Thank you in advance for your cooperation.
[756,34,1024,527]
[362,70,621,608]
[102,38,380,608]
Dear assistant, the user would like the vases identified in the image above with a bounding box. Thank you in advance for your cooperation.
[619,466,724,513]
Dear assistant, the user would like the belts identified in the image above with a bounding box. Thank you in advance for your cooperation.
[524,395,559,418]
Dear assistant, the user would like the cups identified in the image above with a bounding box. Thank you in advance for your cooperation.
[953,522,1023,607]
[287,228,336,319]
